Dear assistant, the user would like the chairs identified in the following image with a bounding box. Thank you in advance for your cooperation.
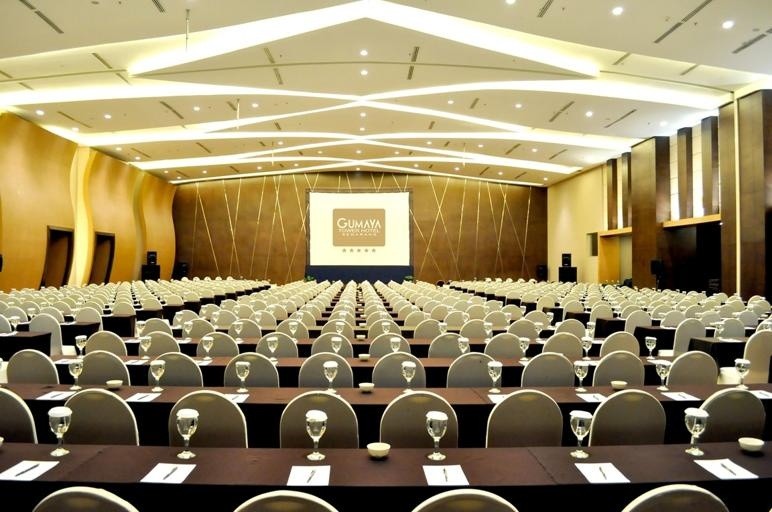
[312,332,355,357]
[1,276,771,341]
[372,351,427,388]
[380,391,458,447]
[197,331,238,355]
[411,487,519,512]
[588,388,666,445]
[64,388,140,446]
[428,333,471,358]
[233,489,340,512]
[740,329,772,384]
[224,352,279,387]
[31,485,141,512]
[599,331,640,357]
[665,350,717,386]
[86,330,128,358]
[485,389,563,446]
[521,352,575,387]
[0,388,39,444]
[256,332,299,357]
[690,388,766,444]
[169,390,249,448]
[148,352,204,387]
[369,332,412,357]
[25,312,66,359]
[446,351,502,387]
[78,350,131,386]
[6,349,60,384]
[298,352,354,387]
[139,331,180,360]
[279,391,360,448]
[620,483,729,512]
[591,350,644,387]
[543,332,586,360]
[484,333,526,362]
[672,318,706,357]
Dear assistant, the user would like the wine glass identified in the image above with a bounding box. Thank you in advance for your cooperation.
[488,361,502,393]
[338,312,346,319]
[504,313,511,328]
[587,322,596,337]
[645,336,657,359]
[381,321,390,334]
[176,409,199,458]
[202,336,213,360]
[401,361,415,394]
[0,279,169,320]
[212,311,220,329]
[655,363,670,391]
[683,407,709,455]
[335,321,344,332]
[10,315,21,334]
[69,361,83,390]
[287,321,299,340]
[139,336,151,359]
[496,301,504,309]
[483,323,493,342]
[581,336,593,360]
[149,360,166,391]
[265,337,279,362]
[546,312,554,328]
[135,321,144,333]
[331,337,342,354]
[235,362,251,394]
[185,320,194,338]
[48,406,72,456]
[483,305,489,313]
[389,338,402,351]
[520,306,526,317]
[380,313,387,318]
[202,305,208,318]
[518,336,529,361]
[424,411,449,462]
[534,322,544,341]
[570,410,592,458]
[423,313,431,319]
[234,305,241,318]
[438,321,447,333]
[462,312,470,323]
[306,409,327,461]
[574,362,588,391]
[716,322,725,338]
[458,338,469,354]
[231,321,243,342]
[322,362,339,393]
[296,312,304,320]
[75,335,86,360]
[175,312,184,327]
[255,312,262,320]
[735,359,751,389]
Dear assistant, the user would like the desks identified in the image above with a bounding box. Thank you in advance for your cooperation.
[0,440,770,492]
[48,348,690,379]
[0,364,772,410]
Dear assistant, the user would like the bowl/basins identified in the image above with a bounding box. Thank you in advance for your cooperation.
[355,334,366,341]
[357,287,368,320]
[611,380,628,389]
[359,323,366,329]
[358,353,371,361]
[366,442,391,459]
[738,437,765,452]
[359,383,375,392]
[105,380,123,390]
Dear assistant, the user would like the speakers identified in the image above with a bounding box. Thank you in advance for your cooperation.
[561,252,571,267]
[147,251,157,265]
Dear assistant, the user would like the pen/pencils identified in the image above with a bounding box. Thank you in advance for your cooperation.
[444,469,447,481]
[16,463,40,476]
[678,394,686,399]
[137,394,149,400]
[231,395,238,401]
[50,393,62,399]
[722,464,737,476]
[164,466,178,479]
[307,470,315,483]
[600,467,607,480]
[593,395,599,400]
